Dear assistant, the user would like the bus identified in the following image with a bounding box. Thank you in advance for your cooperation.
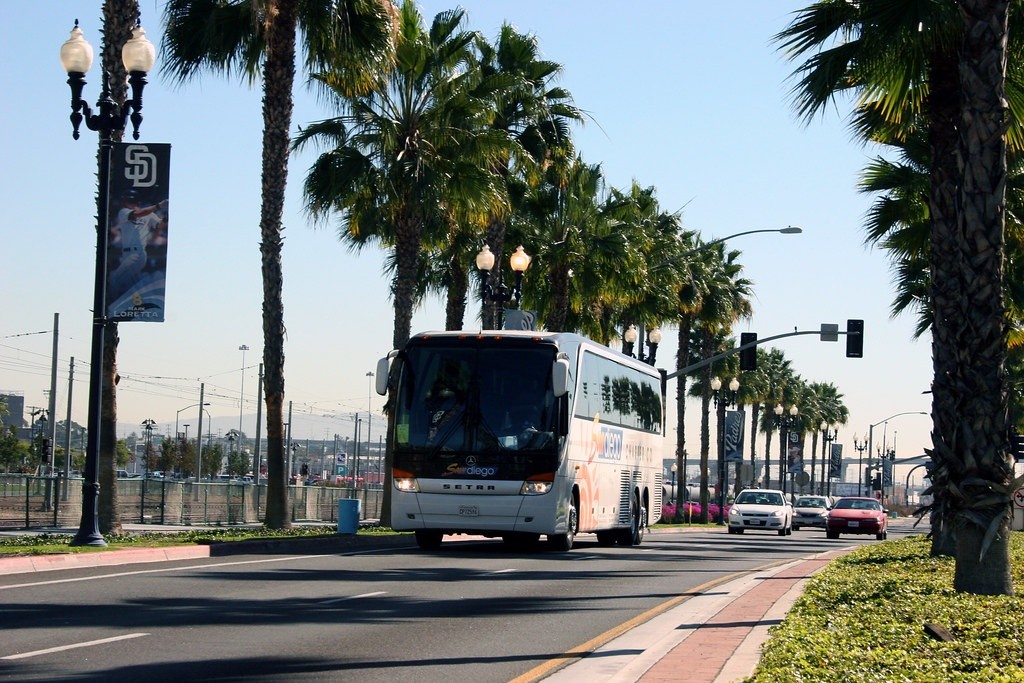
[376,329,663,552]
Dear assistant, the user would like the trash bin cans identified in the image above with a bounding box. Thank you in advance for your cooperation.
[337,498,361,534]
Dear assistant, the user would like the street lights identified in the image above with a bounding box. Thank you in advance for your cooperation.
[183,424,190,438]
[365,371,375,484]
[283,422,289,457]
[290,441,302,476]
[142,418,157,479]
[225,430,239,478]
[59,14,154,547]
[876,441,892,507]
[174,402,211,479]
[819,420,840,497]
[670,462,677,504]
[32,407,49,458]
[773,402,799,497]
[638,224,803,363]
[475,244,533,332]
[867,412,928,496]
[711,375,739,527]
[238,345,250,445]
[853,431,870,497]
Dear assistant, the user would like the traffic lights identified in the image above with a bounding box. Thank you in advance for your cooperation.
[869,475,874,487]
[42,438,52,463]
[890,450,896,461]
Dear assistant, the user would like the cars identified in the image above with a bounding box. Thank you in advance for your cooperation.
[40,466,82,480]
[116,470,180,479]
[827,496,889,541]
[728,488,793,536]
[790,495,833,531]
[214,474,323,486]
